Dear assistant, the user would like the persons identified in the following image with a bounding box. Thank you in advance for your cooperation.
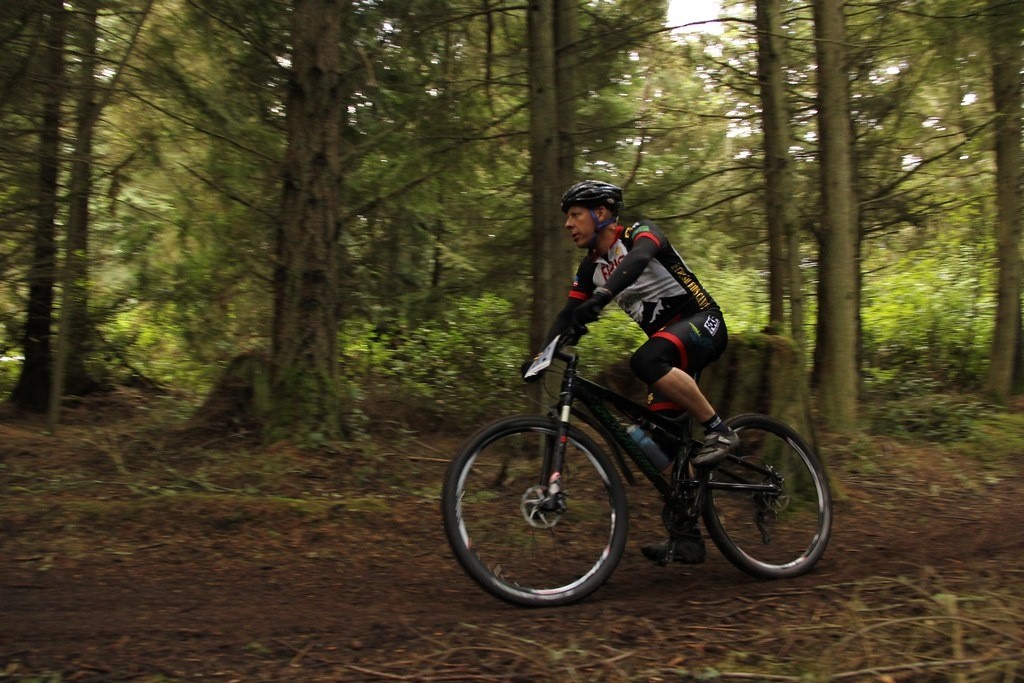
[519,179,741,564]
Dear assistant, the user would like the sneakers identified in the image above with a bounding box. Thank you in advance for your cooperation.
[691,429,741,466]
[640,539,707,565]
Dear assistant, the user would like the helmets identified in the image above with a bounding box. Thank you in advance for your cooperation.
[560,180,624,214]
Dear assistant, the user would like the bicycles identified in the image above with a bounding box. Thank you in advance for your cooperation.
[440,307,833,607]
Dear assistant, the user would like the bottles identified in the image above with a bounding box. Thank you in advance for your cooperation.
[626,425,669,472]
